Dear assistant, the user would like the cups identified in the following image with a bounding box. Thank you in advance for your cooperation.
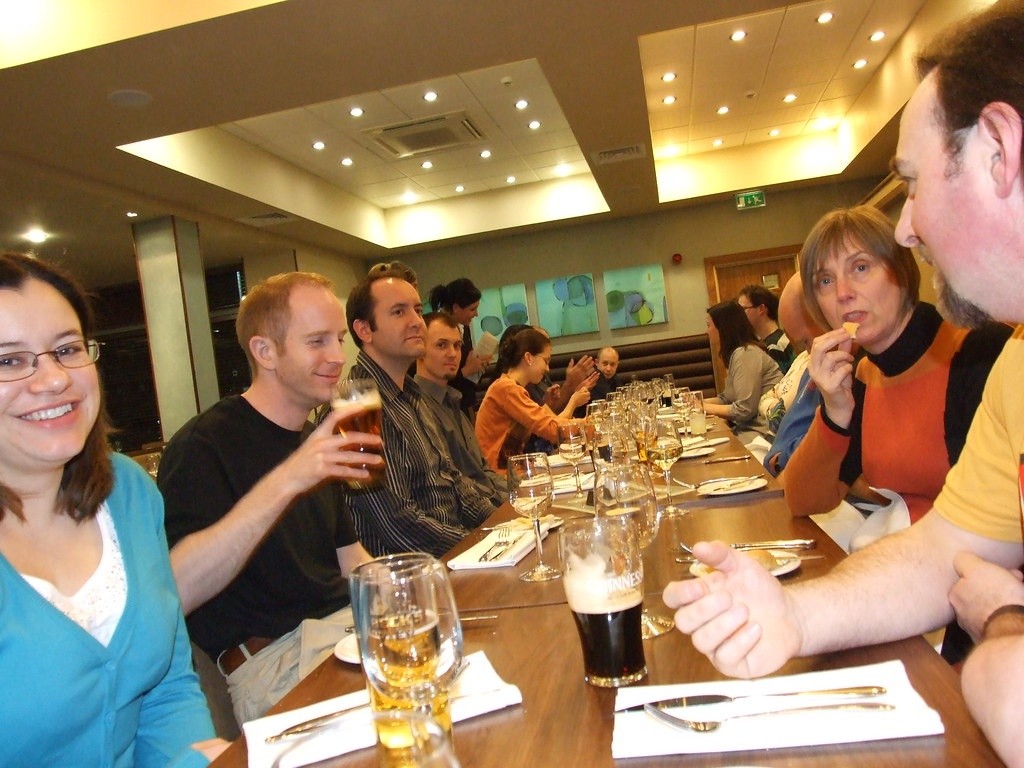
[558,516,649,689]
[327,377,388,491]
[684,391,707,438]
[349,553,455,767]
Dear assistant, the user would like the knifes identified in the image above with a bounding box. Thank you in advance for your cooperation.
[696,473,764,497]
[611,686,888,715]
[489,531,527,562]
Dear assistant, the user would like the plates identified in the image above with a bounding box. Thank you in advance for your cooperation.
[658,397,713,433]
[697,477,768,495]
[334,632,362,666]
[679,447,716,458]
[688,550,801,578]
[496,516,564,530]
[536,454,569,467]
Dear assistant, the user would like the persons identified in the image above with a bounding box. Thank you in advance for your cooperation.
[661,0,1024,767]
[476,323,620,480]
[156,259,522,735]
[1,254,235,768]
[424,277,494,423]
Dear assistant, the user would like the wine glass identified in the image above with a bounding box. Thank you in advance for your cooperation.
[557,374,689,640]
[507,452,562,582]
[357,558,465,767]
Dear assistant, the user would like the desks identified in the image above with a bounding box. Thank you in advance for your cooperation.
[204,416,1006,768]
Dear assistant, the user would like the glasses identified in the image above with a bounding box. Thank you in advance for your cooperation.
[521,351,551,364]
[740,304,767,312]
[0,338,106,382]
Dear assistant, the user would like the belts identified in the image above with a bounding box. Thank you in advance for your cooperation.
[219,636,275,675]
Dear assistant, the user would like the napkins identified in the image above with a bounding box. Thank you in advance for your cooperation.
[299,618,353,682]
[446,523,548,571]
[612,659,945,761]
[241,650,522,768]
[808,487,946,655]
[552,472,594,493]
[744,434,773,465]
[680,437,728,448]
[533,452,592,467]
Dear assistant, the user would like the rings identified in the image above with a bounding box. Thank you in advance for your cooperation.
[590,385,593,389]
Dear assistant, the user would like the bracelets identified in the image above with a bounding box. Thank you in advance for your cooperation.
[973,604,1024,640]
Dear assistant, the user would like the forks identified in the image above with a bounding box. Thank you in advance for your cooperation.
[672,477,749,489]
[642,703,895,733]
[555,474,595,490]
[479,527,511,562]
[679,541,810,555]
[280,654,463,735]
